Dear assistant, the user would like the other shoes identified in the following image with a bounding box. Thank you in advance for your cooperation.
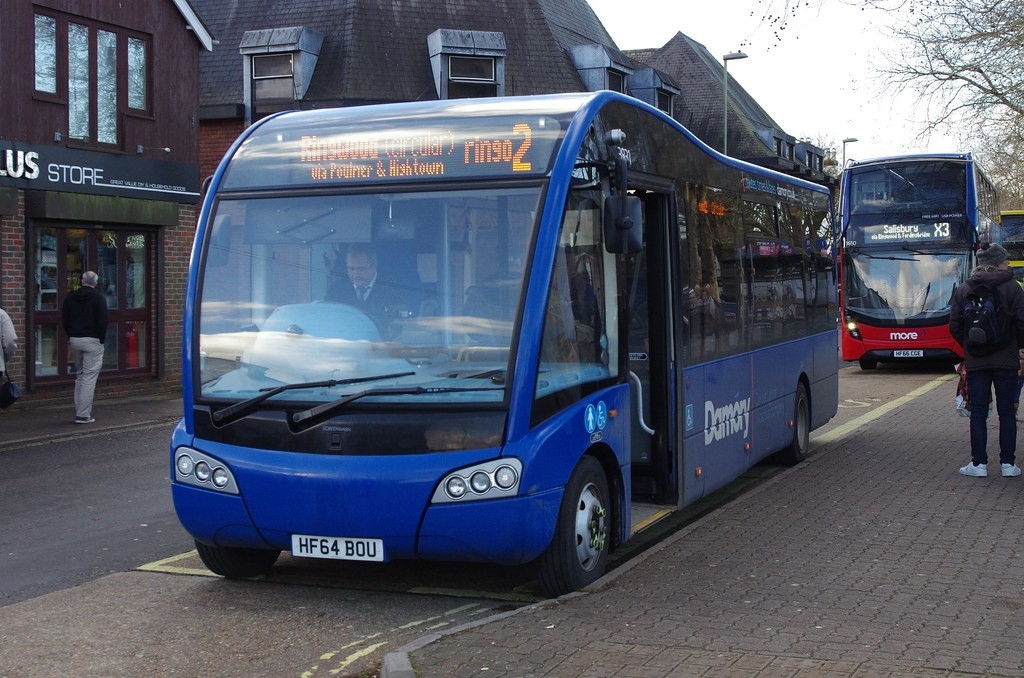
[74,416,95,423]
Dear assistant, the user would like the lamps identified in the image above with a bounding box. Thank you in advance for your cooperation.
[54,132,90,143]
[136,144,171,155]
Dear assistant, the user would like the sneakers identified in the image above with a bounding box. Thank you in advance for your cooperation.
[1001,463,1021,476]
[959,461,987,477]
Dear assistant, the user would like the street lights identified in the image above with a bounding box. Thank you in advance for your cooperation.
[723,50,748,156]
[843,138,858,169]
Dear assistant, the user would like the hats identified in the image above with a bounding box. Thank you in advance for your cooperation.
[975,241,1010,267]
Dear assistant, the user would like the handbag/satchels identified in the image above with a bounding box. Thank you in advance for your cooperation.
[954,361,993,418]
[0,370,22,409]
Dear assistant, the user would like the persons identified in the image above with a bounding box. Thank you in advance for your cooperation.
[324,248,398,341]
[0,308,18,385]
[949,242,1024,477]
[62,271,107,423]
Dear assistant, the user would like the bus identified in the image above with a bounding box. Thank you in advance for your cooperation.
[169,90,840,605]
[735,239,825,303]
[839,152,1001,374]
[1000,210,1024,289]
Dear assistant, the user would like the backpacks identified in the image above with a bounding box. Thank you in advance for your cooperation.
[963,278,1011,357]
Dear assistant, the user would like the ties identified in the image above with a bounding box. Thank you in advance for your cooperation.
[357,287,370,304]
